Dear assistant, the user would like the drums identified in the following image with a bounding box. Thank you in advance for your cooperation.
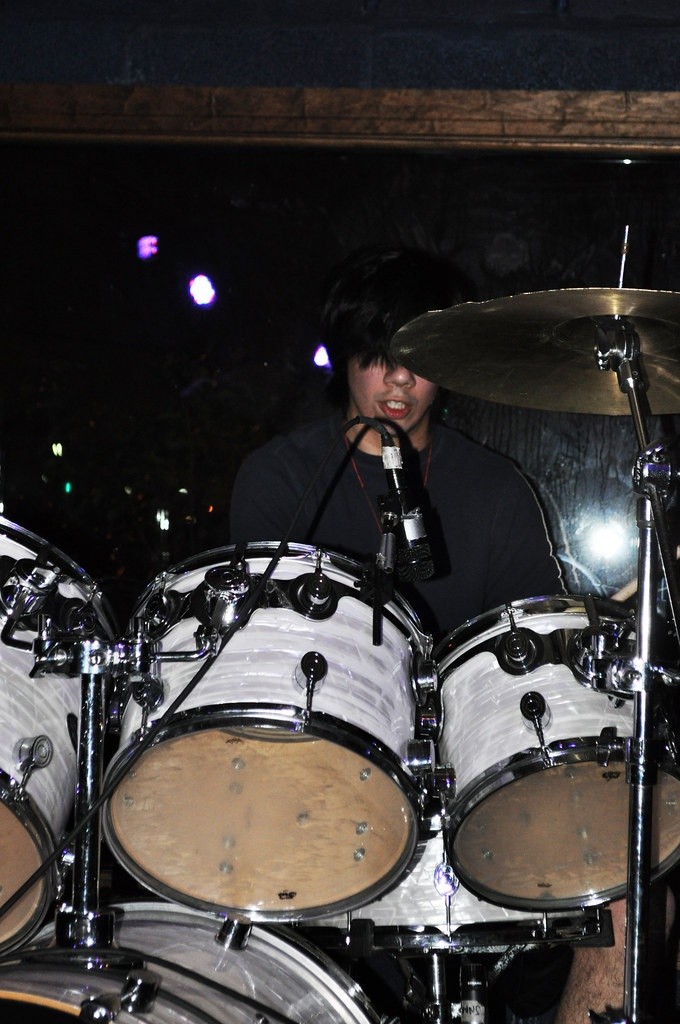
[1,515,125,961]
[2,903,393,1024]
[265,771,615,961]
[101,540,429,927]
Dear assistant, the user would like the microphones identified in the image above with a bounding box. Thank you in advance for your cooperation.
[378,430,436,583]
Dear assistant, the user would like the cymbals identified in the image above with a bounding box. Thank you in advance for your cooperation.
[432,590,679,911]
[385,287,679,418]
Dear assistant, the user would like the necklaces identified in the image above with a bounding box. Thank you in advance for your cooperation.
[226,243,674,1024]
[336,412,433,579]
